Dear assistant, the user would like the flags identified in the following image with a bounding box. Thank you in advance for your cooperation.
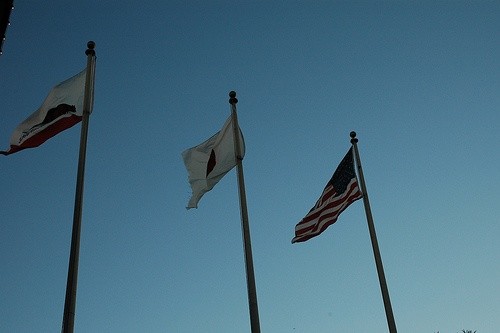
[0,57,96,155]
[180,112,246,210]
[291,146,364,243]
